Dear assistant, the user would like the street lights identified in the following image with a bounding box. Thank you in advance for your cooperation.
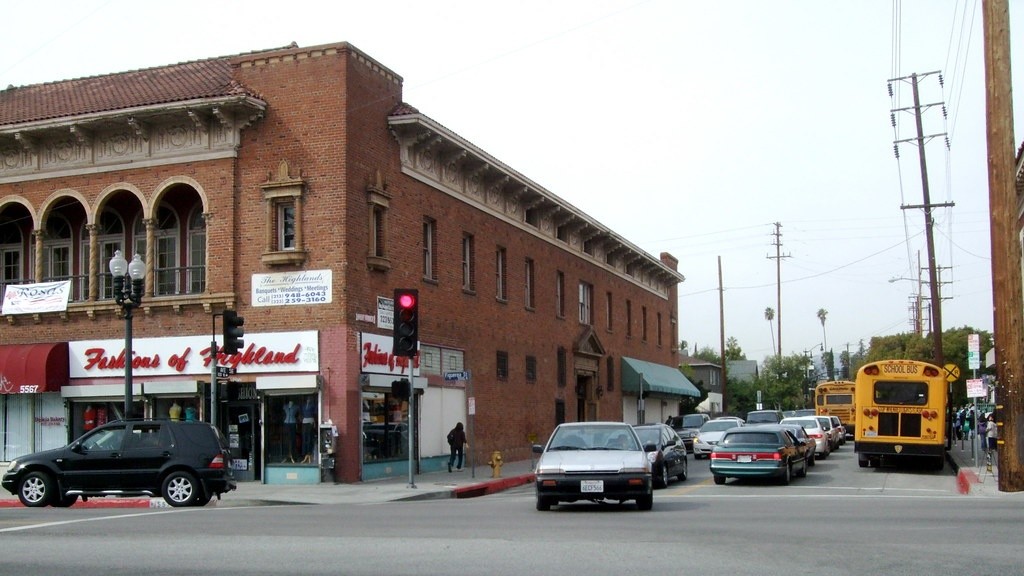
[109,250,146,417]
[889,278,930,287]
[804,343,823,403]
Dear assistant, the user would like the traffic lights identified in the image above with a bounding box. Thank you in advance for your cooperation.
[223,310,244,355]
[393,288,418,357]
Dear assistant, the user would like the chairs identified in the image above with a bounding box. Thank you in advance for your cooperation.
[605,439,629,450]
[558,434,583,448]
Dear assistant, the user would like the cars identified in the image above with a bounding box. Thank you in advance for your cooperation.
[532,421,653,511]
[631,409,846,485]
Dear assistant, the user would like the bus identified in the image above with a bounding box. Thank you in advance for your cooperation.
[815,380,856,438]
[855,360,946,471]
[361,393,408,423]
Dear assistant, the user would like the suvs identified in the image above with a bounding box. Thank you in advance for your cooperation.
[1,417,237,507]
[664,413,710,450]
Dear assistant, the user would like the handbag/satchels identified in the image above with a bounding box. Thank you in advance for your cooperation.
[963,421,969,433]
[448,429,454,446]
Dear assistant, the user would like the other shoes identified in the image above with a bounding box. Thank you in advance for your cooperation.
[457,468,463,472]
[448,465,452,473]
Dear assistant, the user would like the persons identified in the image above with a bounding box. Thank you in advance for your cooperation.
[300,396,318,464]
[184,408,197,422]
[83,406,96,434]
[168,402,182,422]
[446,422,469,473]
[97,404,108,427]
[281,401,301,463]
[948,402,997,455]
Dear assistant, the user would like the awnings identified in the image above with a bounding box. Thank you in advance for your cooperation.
[0,342,70,394]
[620,357,701,397]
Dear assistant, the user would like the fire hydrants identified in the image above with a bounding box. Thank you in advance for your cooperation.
[488,451,504,478]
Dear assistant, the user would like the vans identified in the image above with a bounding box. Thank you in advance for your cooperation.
[365,422,408,440]
